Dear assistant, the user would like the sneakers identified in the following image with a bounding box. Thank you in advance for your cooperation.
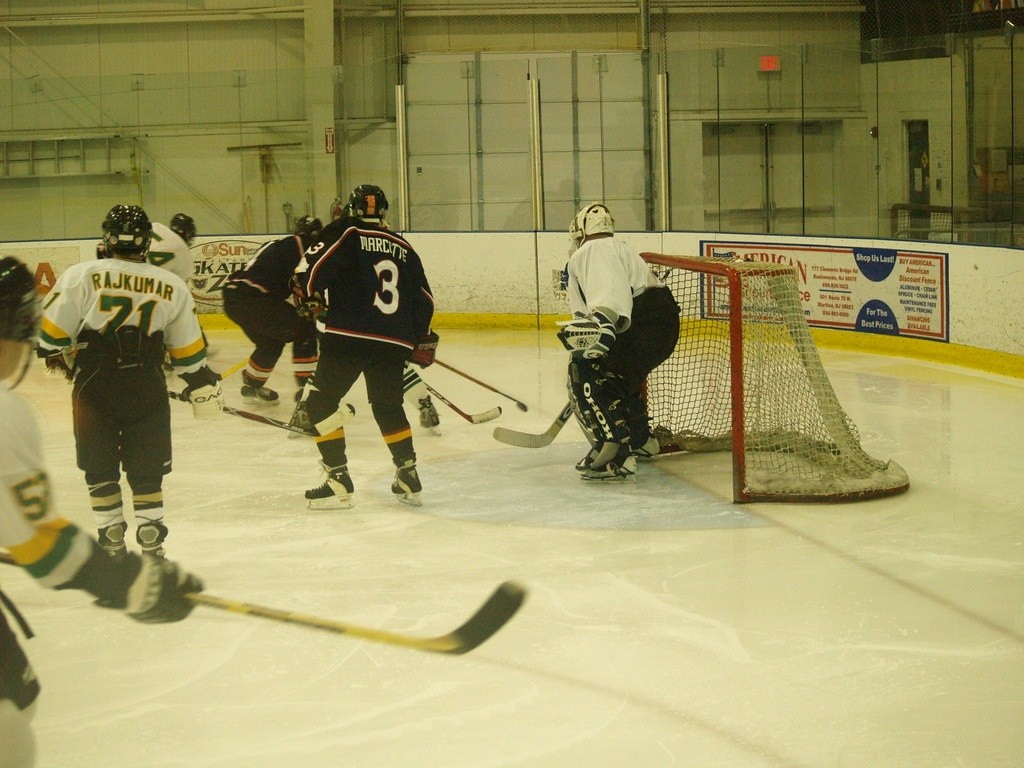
[630,426,660,463]
[304,459,355,509]
[418,396,440,429]
[575,437,638,484]
[390,451,423,506]
[140,543,167,559]
[99,542,127,559]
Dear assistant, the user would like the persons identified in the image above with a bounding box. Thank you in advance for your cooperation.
[0,257,204,768]
[37,204,222,567]
[288,184,440,500]
[220,215,324,401]
[554,202,681,478]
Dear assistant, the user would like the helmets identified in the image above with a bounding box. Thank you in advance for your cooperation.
[349,184,388,224]
[0,256,41,339]
[294,216,323,243]
[568,219,580,248]
[169,213,196,245]
[575,201,614,244]
[100,202,153,261]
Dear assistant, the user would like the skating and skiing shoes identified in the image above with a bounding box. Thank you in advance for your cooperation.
[240,369,281,407]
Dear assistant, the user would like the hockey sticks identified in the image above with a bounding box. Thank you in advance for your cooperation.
[45,358,357,437]
[423,380,502,424]
[291,285,528,411]
[0,552,528,660]
[203,357,250,381]
[493,269,671,448]
